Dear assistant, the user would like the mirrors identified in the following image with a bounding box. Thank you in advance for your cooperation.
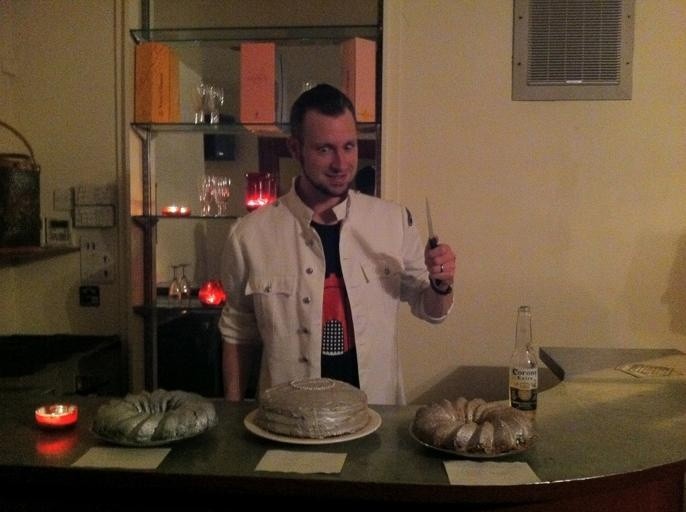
[149,2,379,311]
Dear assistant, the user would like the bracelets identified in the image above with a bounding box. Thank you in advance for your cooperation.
[428,273,453,296]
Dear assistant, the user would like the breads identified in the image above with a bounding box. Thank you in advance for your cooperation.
[94,387,217,443]
[260,378,369,440]
[414,396,532,455]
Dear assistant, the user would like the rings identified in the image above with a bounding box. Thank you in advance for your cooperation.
[441,264,443,271]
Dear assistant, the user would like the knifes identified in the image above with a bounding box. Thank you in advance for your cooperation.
[422,197,450,286]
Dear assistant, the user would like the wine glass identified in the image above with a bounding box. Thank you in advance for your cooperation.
[198,171,232,216]
[167,262,191,303]
[188,81,224,125]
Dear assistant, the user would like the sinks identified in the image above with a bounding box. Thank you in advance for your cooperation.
[0,334,121,391]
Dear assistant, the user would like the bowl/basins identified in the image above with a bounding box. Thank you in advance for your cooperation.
[35,432,78,456]
[34,405,79,429]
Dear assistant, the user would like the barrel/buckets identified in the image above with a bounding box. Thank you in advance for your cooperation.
[1,121,42,245]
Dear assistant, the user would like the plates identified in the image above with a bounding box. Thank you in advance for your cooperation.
[243,401,381,446]
[87,422,185,448]
[407,421,519,457]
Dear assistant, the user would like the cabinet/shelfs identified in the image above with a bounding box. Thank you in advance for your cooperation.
[128,1,381,400]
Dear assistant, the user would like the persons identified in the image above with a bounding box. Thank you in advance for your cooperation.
[216,82,456,408]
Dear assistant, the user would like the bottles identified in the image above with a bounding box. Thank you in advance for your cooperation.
[509,304,540,421]
[197,278,222,308]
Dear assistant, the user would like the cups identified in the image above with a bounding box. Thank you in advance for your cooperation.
[301,80,319,95]
[245,172,277,213]
